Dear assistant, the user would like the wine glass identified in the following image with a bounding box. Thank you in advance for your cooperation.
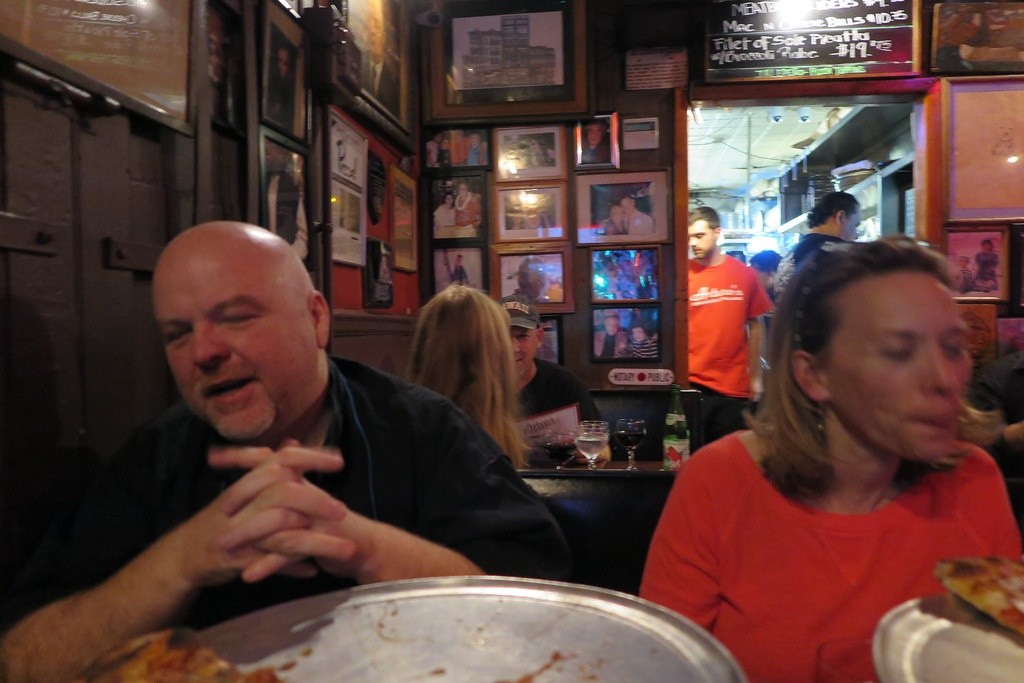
[543,430,576,469]
[575,420,610,469]
[615,418,647,469]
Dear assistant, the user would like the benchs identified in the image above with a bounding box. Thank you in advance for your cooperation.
[582,386,705,466]
[495,466,684,598]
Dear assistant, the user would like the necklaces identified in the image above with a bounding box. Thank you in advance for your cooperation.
[455,192,470,211]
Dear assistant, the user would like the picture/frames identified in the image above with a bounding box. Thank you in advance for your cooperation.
[573,110,622,172]
[939,74,1024,225]
[929,2,1024,73]
[258,0,597,366]
[587,244,664,306]
[592,306,664,364]
[574,168,671,244]
[941,224,1011,303]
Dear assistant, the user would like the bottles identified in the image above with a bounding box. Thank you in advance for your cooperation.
[663,384,690,472]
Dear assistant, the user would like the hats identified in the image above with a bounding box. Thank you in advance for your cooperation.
[496,296,542,331]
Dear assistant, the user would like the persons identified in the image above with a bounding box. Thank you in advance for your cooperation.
[406,192,1024,531]
[974,238,1000,288]
[267,0,661,361]
[935,4,1024,65]
[0,221,575,683]
[636,233,1024,683]
[949,255,990,293]
